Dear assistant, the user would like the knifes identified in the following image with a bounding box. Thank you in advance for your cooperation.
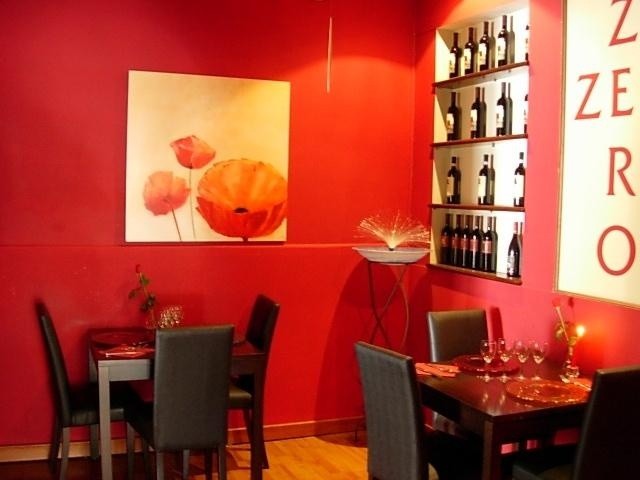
[103,349,148,358]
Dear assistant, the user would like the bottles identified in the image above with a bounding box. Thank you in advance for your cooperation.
[445,156,462,204]
[506,223,522,278]
[476,154,489,206]
[523,19,528,62]
[439,214,496,274]
[514,150,526,207]
[445,15,507,77]
[447,82,509,140]
[522,86,527,136]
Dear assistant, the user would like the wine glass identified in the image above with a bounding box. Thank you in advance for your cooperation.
[480,337,548,382]
[554,319,584,382]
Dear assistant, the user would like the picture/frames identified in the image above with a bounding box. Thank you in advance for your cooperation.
[552,1,640,311]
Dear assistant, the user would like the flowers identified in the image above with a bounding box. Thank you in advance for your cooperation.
[552,297,579,372]
[128,263,158,323]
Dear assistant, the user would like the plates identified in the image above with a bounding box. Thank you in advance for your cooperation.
[505,377,590,404]
[91,329,152,347]
[233,330,247,348]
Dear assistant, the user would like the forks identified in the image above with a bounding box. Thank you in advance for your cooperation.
[414,360,462,382]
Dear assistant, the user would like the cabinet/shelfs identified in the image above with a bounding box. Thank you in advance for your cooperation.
[429,1,528,286]
